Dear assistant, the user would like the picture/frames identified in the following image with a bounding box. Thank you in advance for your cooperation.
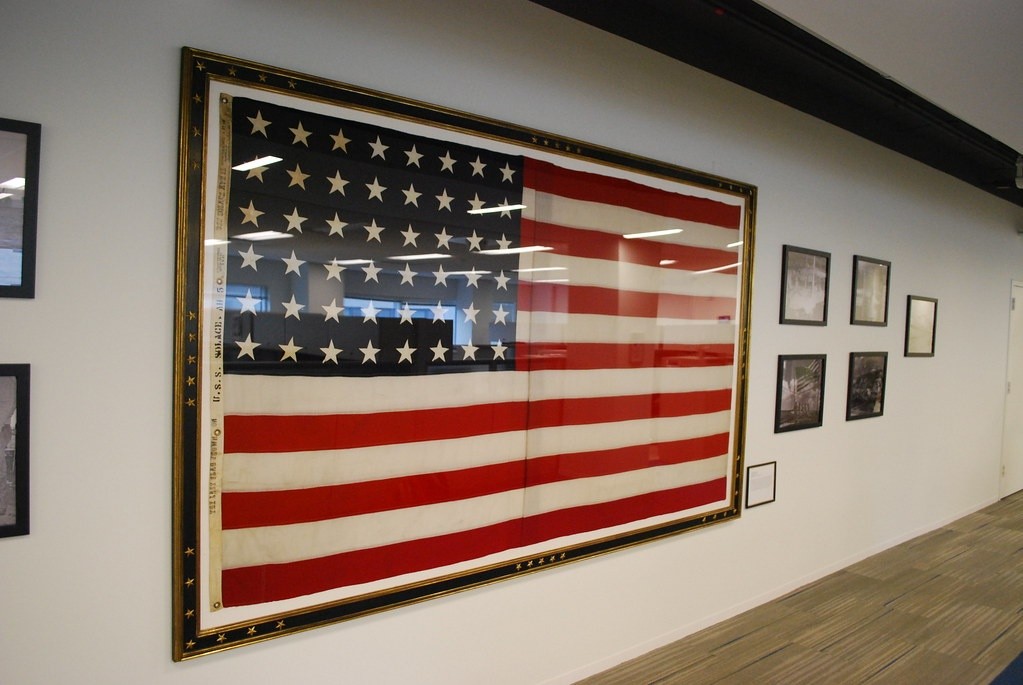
[0,363,30,539]
[172,47,758,662]
[904,295,938,357]
[850,255,891,327]
[774,354,827,433]
[845,352,888,421]
[0,117,42,299]
[779,245,831,326]
[746,461,776,508]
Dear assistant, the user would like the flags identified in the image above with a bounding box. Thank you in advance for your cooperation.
[208,92,743,613]
